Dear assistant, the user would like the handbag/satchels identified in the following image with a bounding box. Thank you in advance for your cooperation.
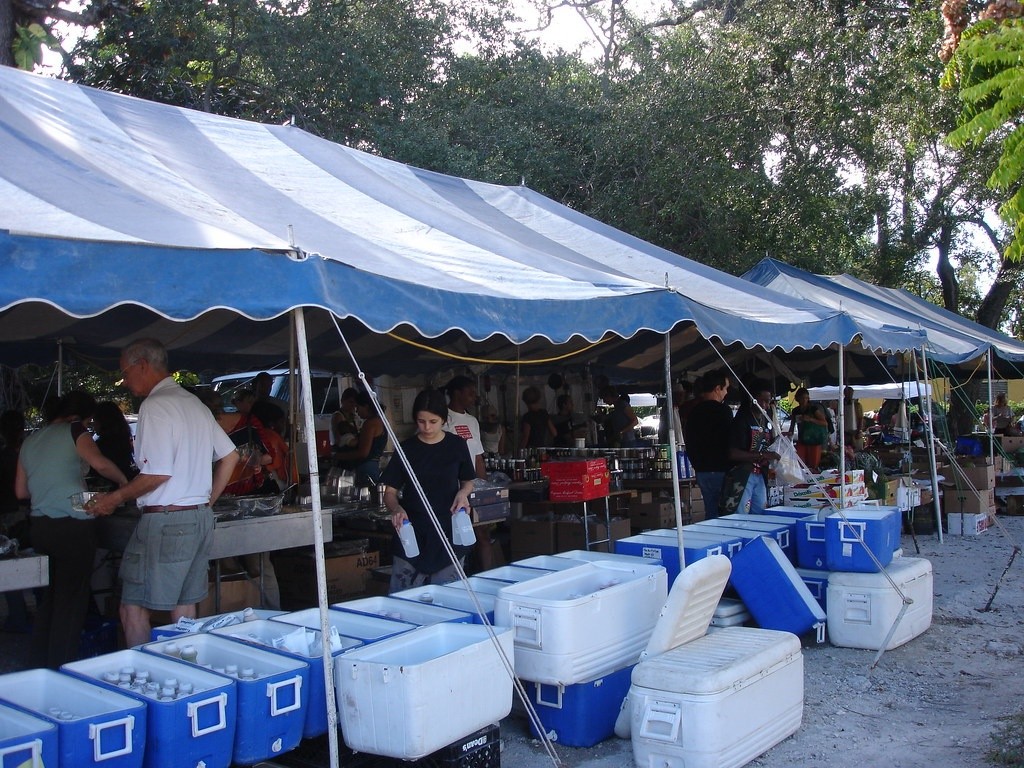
[844,400,857,431]
[767,436,808,484]
[1007,420,1020,436]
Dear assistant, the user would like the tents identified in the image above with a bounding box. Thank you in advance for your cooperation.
[735,258,1024,543]
[0,65,863,768]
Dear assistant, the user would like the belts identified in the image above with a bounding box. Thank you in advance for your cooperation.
[141,502,210,513]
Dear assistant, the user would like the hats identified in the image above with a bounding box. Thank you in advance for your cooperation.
[480,404,498,418]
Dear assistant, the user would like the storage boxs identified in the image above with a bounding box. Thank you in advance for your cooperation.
[58,647,238,768]
[141,433,1024,768]
[0,700,59,768]
[0,668,148,768]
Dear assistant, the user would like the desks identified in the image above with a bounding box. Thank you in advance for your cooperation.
[0,508,332,614]
[528,490,632,553]
[622,477,697,525]
[333,517,508,540]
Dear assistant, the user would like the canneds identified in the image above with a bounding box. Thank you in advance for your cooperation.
[575,438,585,448]
[485,458,542,480]
[520,448,673,480]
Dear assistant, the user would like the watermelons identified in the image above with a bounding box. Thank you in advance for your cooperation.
[961,461,976,468]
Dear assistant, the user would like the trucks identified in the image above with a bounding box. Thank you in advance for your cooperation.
[211,367,350,477]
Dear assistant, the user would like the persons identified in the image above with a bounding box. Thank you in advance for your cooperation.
[442,377,492,570]
[782,387,1015,474]
[183,373,311,611]
[85,338,240,648]
[658,371,781,520]
[0,410,29,635]
[479,386,638,457]
[329,388,388,481]
[83,401,140,634]
[15,391,129,671]
[1016,415,1024,437]
[384,388,477,595]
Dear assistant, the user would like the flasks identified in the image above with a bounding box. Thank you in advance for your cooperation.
[377,482,388,508]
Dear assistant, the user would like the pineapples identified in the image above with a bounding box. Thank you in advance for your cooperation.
[866,469,889,499]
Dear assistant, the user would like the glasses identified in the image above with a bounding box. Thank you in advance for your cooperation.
[120,358,149,383]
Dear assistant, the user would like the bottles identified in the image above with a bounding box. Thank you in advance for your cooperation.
[420,592,432,604]
[484,448,531,469]
[399,520,419,558]
[456,509,477,546]
[379,610,400,619]
[452,509,464,545]
[49,707,79,720]
[105,642,266,702]
